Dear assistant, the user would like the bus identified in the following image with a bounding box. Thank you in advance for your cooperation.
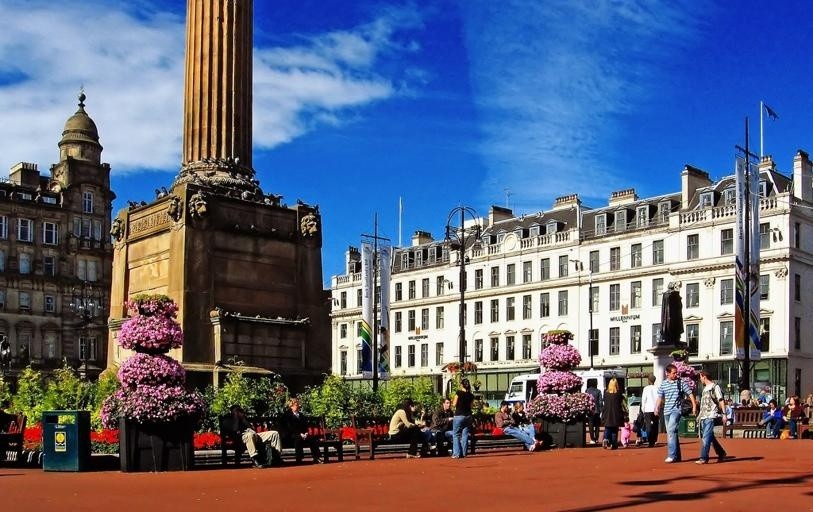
[500,369,628,425]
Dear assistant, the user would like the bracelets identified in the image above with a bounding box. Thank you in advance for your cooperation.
[722,412,727,415]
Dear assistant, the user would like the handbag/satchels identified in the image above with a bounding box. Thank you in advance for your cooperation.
[681,399,693,415]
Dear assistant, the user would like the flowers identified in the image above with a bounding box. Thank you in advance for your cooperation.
[100,294,205,430]
[526,329,596,421]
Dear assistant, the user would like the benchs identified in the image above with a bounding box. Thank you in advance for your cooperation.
[350,414,432,461]
[218,415,344,465]
[0,415,25,466]
[469,413,527,453]
[722,408,792,440]
[796,407,813,438]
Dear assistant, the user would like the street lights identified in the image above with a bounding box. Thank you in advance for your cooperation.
[442,205,485,384]
[0,334,11,383]
[67,280,103,381]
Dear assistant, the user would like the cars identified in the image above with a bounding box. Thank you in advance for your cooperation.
[626,399,642,423]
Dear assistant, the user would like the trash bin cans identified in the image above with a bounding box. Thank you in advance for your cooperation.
[43,410,92,472]
[680,415,698,438]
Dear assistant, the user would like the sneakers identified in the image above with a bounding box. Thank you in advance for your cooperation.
[665,458,674,462]
[695,459,705,464]
[406,453,421,459]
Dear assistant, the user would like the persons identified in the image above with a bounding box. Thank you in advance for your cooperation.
[227,405,289,469]
[510,402,536,449]
[386,397,427,458]
[428,395,463,456]
[493,400,543,452]
[450,378,474,459]
[585,369,813,448]
[280,397,326,465]
[693,370,728,466]
[652,365,695,463]
[407,400,440,457]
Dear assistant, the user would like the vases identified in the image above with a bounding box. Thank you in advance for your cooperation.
[542,416,586,449]
[119,419,196,472]
